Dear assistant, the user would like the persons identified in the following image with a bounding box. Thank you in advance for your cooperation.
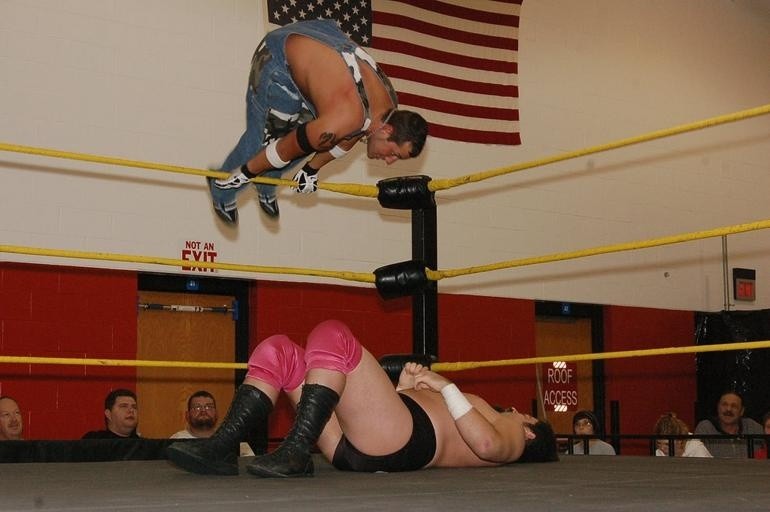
[81,388,140,439]
[166,318,557,478]
[652,411,713,457]
[693,392,764,458]
[565,409,617,456]
[169,390,219,439]
[206,18,428,223]
[0,396,26,440]
[751,413,770,460]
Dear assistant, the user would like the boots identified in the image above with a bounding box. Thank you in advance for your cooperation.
[164,384,271,475]
[247,386,339,477]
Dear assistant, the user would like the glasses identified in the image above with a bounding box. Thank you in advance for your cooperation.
[573,422,590,428]
[189,406,214,410]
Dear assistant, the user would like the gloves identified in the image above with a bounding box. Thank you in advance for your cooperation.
[212,164,255,189]
[290,164,320,194]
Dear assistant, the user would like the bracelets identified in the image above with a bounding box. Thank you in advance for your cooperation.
[440,382,473,421]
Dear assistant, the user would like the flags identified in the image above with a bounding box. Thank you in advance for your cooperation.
[262,0,524,147]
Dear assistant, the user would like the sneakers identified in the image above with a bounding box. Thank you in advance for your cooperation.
[259,191,280,217]
[205,168,237,222]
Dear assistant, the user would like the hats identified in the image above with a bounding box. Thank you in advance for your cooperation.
[573,411,599,431]
